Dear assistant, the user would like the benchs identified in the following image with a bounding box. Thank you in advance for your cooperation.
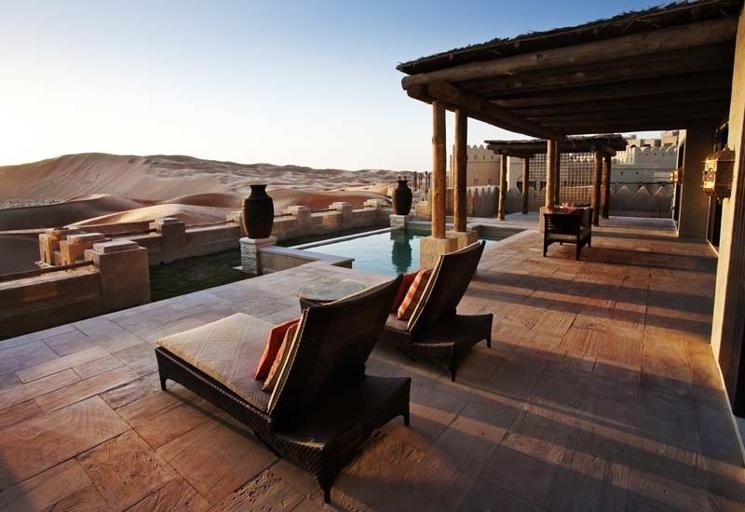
[541,204,593,260]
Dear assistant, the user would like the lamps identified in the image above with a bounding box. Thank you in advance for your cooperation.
[698,144,736,204]
